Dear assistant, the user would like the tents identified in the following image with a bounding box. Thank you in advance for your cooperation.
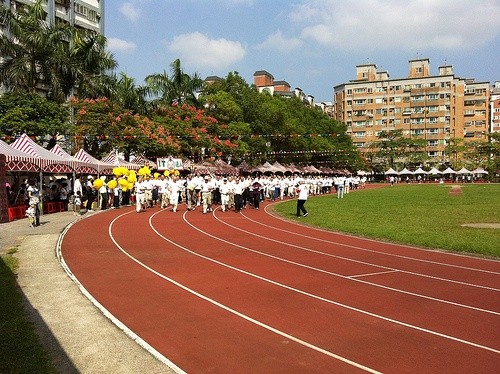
[0,134,490,174]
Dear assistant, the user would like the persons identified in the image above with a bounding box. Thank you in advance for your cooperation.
[7,173,490,227]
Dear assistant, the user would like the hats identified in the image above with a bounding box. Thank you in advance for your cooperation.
[222,177,227,180]
[204,176,208,179]
[236,178,239,180]
[87,175,92,179]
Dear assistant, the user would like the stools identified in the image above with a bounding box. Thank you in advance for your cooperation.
[2,202,65,222]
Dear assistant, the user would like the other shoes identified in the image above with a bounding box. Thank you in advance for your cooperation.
[135,191,345,221]
